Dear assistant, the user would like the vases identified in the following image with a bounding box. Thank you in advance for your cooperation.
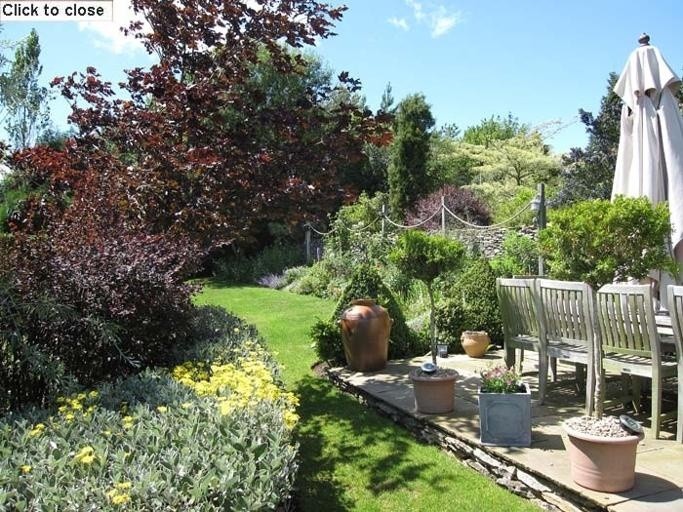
[478,381,532,449]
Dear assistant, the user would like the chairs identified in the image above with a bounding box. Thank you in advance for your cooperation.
[494,271,682,440]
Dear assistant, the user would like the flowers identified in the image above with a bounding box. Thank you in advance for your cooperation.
[475,360,524,393]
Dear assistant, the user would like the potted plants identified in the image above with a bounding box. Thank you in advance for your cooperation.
[324,216,394,370]
[535,196,682,491]
[434,244,509,358]
[387,226,468,416]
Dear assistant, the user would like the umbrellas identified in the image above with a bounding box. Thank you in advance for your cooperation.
[609,32,683,314]
